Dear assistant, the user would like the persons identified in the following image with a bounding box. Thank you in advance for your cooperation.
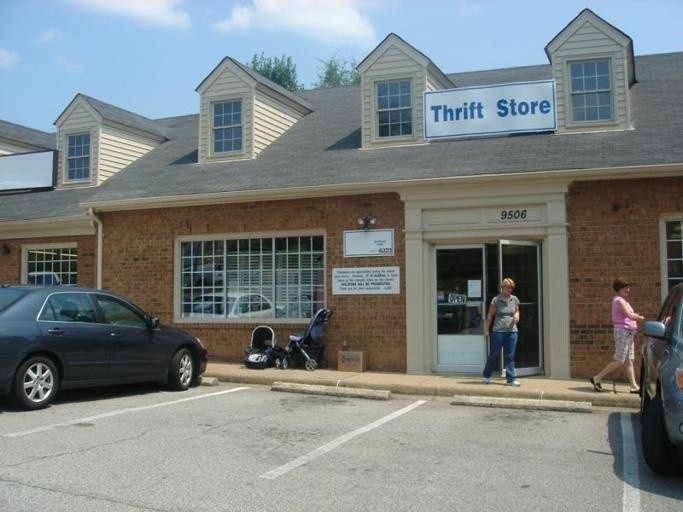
[590,277,645,393]
[482,278,521,386]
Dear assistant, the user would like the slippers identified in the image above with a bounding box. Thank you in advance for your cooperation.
[590,377,602,391]
[630,386,641,393]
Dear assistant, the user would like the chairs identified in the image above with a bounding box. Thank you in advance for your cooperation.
[57,301,79,321]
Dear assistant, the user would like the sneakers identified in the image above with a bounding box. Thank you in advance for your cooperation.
[507,380,521,386]
[483,377,489,383]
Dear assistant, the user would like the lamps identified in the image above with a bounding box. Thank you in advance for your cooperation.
[357,214,377,229]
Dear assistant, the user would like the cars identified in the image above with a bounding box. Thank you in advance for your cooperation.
[0,284,209,409]
[637,281,683,478]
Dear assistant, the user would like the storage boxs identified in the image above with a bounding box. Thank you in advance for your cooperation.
[336,349,367,372]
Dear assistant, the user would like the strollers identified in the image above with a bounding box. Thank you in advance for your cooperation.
[274,307,334,371]
